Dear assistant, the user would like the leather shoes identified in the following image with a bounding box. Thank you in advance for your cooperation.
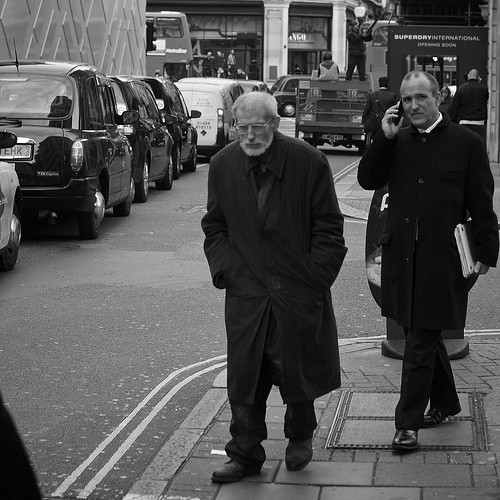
[423,401,461,427]
[391,430,418,451]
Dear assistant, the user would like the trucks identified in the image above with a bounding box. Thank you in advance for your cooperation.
[295,70,373,153]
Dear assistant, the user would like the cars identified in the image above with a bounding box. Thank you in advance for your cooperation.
[270,75,320,117]
[0,38,140,273]
[108,76,203,204]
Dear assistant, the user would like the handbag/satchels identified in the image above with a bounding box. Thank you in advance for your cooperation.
[364,91,382,132]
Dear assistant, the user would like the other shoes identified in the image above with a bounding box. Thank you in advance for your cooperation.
[211,460,261,484]
[285,437,312,471]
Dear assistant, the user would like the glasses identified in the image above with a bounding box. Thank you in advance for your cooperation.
[235,116,274,134]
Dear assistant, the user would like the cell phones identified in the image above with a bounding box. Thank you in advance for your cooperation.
[392,98,405,126]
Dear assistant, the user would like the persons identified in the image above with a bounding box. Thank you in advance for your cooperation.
[202,49,238,79]
[361,76,398,144]
[164,27,171,37]
[344,18,373,82]
[438,68,489,141]
[200,91,351,485]
[315,51,340,81]
[354,69,500,455]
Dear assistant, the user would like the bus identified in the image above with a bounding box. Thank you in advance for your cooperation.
[145,11,194,82]
